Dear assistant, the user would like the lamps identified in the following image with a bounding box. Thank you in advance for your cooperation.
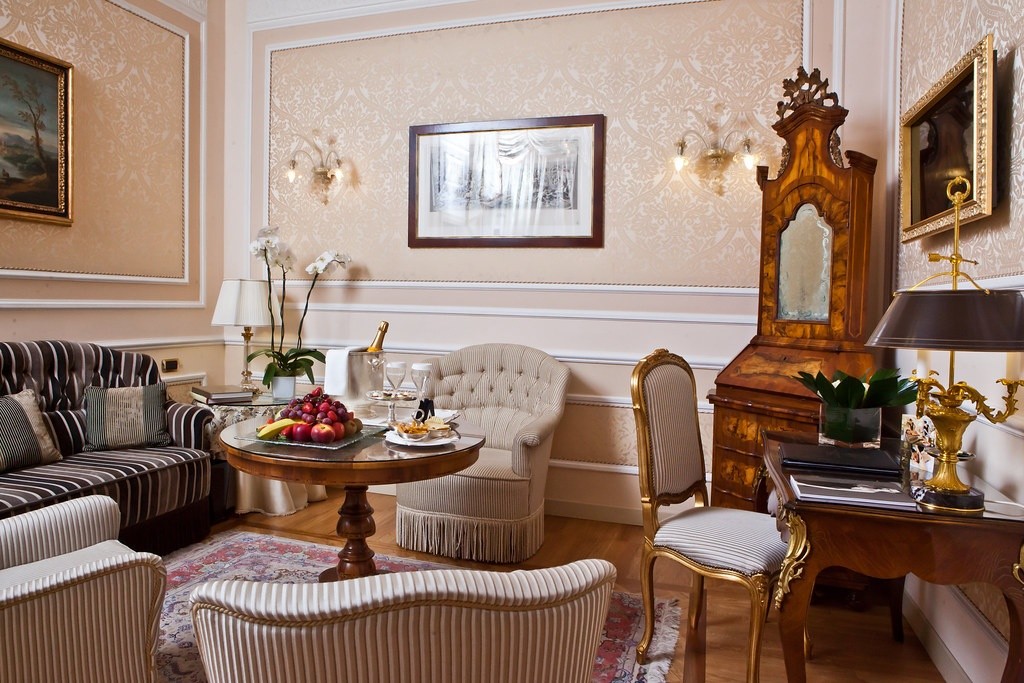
[288,150,344,205]
[673,130,755,196]
[864,176,1024,514]
[211,279,282,396]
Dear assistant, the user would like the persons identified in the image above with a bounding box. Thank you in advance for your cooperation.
[795,479,903,494]
[906,419,931,464]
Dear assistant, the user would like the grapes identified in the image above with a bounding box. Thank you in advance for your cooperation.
[281,394,348,424]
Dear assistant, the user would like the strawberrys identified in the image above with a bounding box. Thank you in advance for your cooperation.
[302,387,338,421]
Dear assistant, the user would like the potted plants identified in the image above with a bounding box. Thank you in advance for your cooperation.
[792,366,933,449]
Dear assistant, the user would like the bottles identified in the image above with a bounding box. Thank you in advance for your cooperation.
[366,320,389,353]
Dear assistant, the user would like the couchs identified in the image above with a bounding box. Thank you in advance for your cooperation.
[0,340,214,557]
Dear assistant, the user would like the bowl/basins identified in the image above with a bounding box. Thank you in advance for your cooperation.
[428,428,451,438]
[397,431,429,440]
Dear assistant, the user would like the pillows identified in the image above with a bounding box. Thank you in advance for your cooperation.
[81,381,171,452]
[0,390,64,476]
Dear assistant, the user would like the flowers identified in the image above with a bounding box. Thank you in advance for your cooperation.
[246,224,354,390]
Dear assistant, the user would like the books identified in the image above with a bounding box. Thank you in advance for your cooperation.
[778,442,904,480]
[788,474,916,508]
[190,386,253,404]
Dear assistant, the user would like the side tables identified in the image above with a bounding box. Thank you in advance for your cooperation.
[191,392,329,517]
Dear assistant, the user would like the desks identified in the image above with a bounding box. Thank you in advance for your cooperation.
[218,405,487,583]
[751,427,1024,683]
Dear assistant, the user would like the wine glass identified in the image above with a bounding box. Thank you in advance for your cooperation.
[410,363,434,397]
[385,363,407,398]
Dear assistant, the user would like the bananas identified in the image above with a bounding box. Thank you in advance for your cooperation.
[256,419,313,440]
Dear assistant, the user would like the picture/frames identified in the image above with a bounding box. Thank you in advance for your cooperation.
[407,115,605,249]
[899,33,999,245]
[0,36,75,225]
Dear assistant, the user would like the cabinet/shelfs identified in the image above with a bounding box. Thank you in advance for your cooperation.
[707,64,881,602]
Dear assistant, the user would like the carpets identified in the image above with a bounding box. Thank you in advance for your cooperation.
[155,533,682,683]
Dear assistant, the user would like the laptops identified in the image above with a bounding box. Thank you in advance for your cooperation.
[777,443,903,476]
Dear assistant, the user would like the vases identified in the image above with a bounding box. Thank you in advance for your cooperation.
[272,377,296,400]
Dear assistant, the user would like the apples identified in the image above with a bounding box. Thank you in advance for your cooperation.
[268,416,363,445]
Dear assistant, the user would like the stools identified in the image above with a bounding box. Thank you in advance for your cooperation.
[0,494,167,683]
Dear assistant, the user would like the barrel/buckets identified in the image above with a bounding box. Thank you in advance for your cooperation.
[344,350,384,399]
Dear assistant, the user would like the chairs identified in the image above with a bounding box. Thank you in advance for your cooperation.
[630,349,813,683]
[188,557,616,683]
[396,342,570,563]
